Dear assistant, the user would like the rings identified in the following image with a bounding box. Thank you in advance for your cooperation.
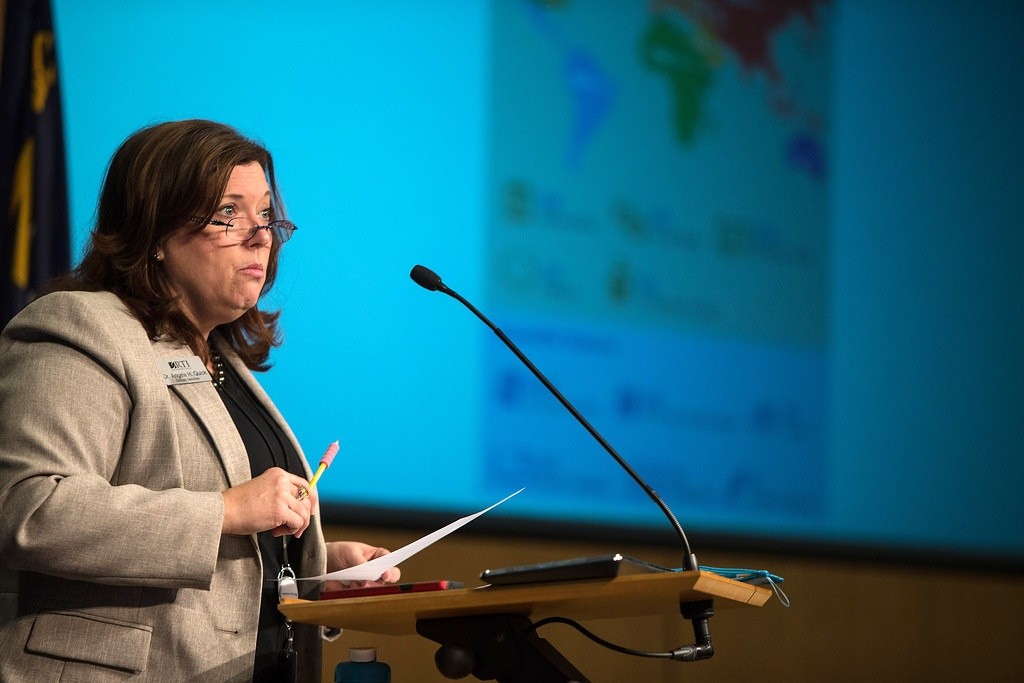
[294,485,309,502]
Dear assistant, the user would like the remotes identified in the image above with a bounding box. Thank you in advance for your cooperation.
[479,554,623,585]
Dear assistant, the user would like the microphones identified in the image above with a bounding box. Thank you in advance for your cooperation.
[409,264,697,571]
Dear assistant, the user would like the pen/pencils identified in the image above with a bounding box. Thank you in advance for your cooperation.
[308,439,342,491]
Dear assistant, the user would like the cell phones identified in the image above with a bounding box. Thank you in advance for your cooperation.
[319,581,464,601]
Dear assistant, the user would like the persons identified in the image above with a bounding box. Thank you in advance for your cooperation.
[0,121,400,683]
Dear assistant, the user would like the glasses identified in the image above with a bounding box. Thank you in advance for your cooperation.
[187,214,298,249]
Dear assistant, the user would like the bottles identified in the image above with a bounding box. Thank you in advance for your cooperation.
[335,647,391,683]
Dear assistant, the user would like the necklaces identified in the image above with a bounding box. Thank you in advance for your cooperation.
[209,345,225,390]
